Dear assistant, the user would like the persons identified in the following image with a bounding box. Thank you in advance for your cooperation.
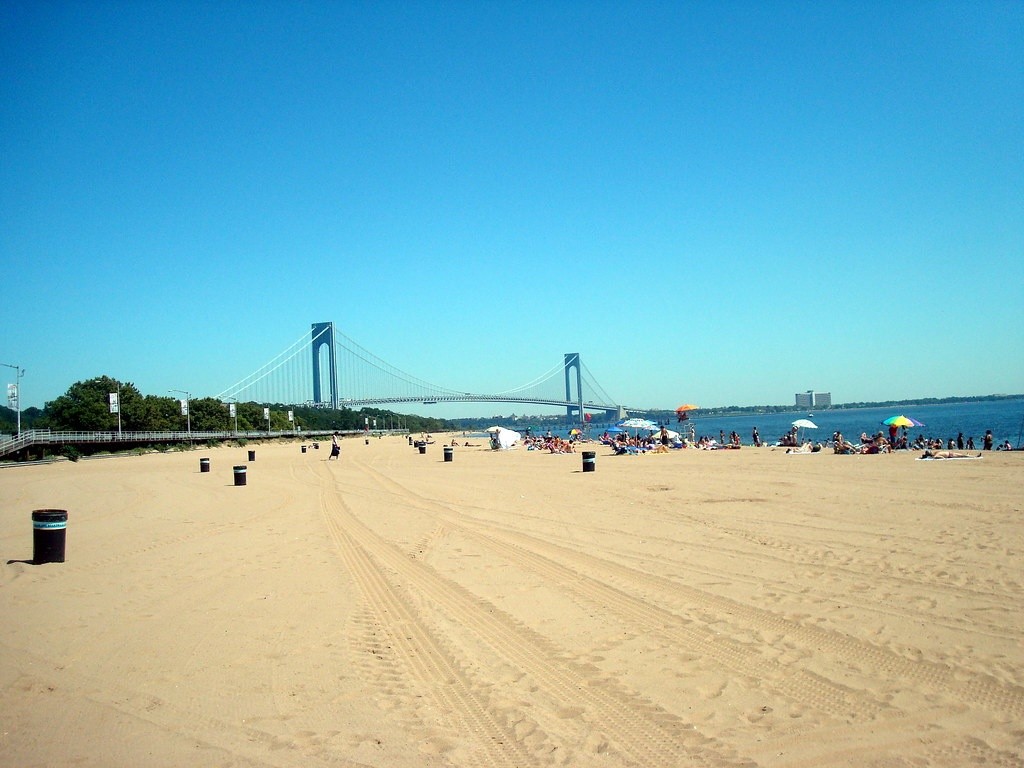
[489,438,502,450]
[966,437,976,450]
[957,433,963,450]
[832,430,892,455]
[996,444,1003,451]
[426,434,431,441]
[924,450,981,459]
[982,430,993,450]
[465,442,469,446]
[569,433,584,445]
[1004,440,1012,451]
[887,425,944,451]
[524,430,577,454]
[777,426,830,453]
[421,432,424,437]
[451,439,459,446]
[329,432,340,460]
[947,438,955,449]
[609,426,768,456]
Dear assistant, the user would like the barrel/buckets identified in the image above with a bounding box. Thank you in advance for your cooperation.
[32,510,68,564]
[406,436,407,439]
[414,441,418,448]
[418,442,427,454]
[248,450,255,461]
[365,440,369,445]
[582,451,596,472]
[379,436,381,439]
[233,466,247,486]
[301,446,306,453]
[409,437,413,445]
[313,443,319,449]
[200,458,209,472]
[444,448,453,461]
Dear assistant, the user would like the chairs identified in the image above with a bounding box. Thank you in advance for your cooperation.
[843,441,864,452]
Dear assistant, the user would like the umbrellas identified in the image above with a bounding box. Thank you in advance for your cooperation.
[792,418,817,444]
[567,429,582,435]
[605,426,623,437]
[676,404,700,418]
[880,415,926,448]
[616,416,661,448]
[484,425,507,443]
[652,429,679,439]
